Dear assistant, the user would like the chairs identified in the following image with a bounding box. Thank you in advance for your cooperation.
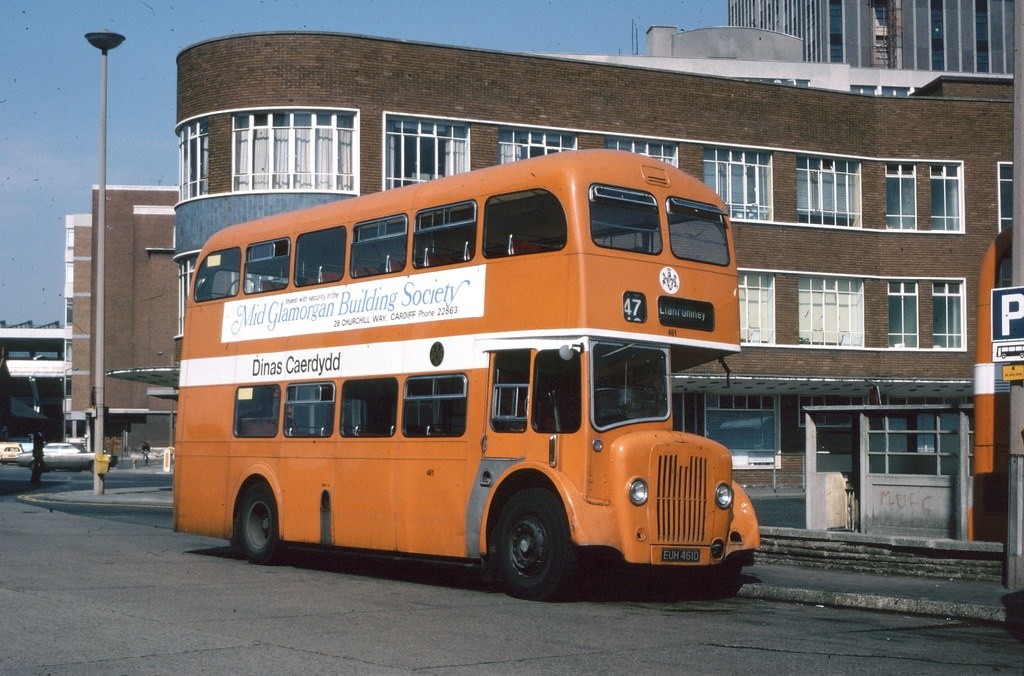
[258,234,545,294]
[286,426,451,437]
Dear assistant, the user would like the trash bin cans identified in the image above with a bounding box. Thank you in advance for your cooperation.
[164,447,175,473]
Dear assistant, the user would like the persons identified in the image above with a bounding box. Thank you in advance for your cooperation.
[31,431,46,483]
[142,441,150,463]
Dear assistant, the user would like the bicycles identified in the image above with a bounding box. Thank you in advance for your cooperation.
[141,451,151,466]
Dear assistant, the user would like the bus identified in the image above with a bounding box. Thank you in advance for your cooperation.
[168,147,761,606]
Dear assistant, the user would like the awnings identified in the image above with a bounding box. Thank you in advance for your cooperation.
[11,396,48,419]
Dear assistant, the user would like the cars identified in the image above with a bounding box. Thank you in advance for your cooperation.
[0,445,25,465]
[15,440,119,474]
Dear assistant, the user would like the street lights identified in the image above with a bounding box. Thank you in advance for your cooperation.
[81,28,130,496]
